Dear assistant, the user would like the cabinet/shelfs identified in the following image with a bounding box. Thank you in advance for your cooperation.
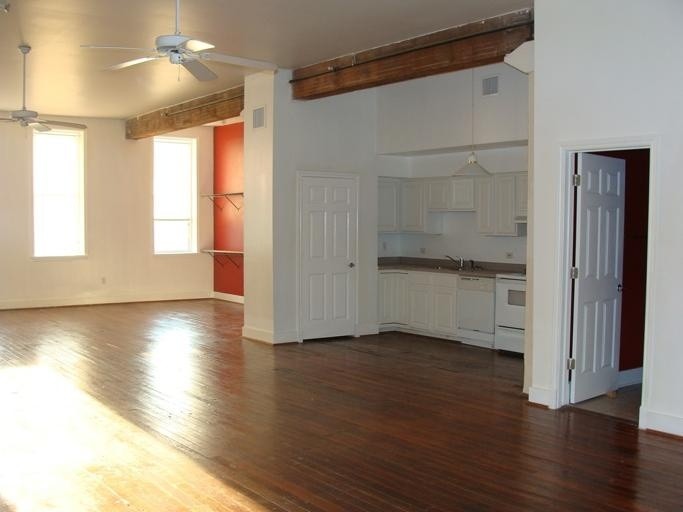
[199,193,243,269]
[475,174,521,238]
[378,175,398,234]
[378,270,459,342]
[513,172,528,224]
[398,176,443,235]
[426,176,475,212]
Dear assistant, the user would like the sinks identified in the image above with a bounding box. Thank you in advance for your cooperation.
[439,264,459,272]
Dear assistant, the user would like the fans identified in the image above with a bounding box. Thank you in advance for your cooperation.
[0,45,87,133]
[78,0,279,82]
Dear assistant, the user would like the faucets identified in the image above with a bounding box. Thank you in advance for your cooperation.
[469,259,475,272]
[445,254,465,271]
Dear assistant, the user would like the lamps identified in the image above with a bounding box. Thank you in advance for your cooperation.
[466,66,478,164]
